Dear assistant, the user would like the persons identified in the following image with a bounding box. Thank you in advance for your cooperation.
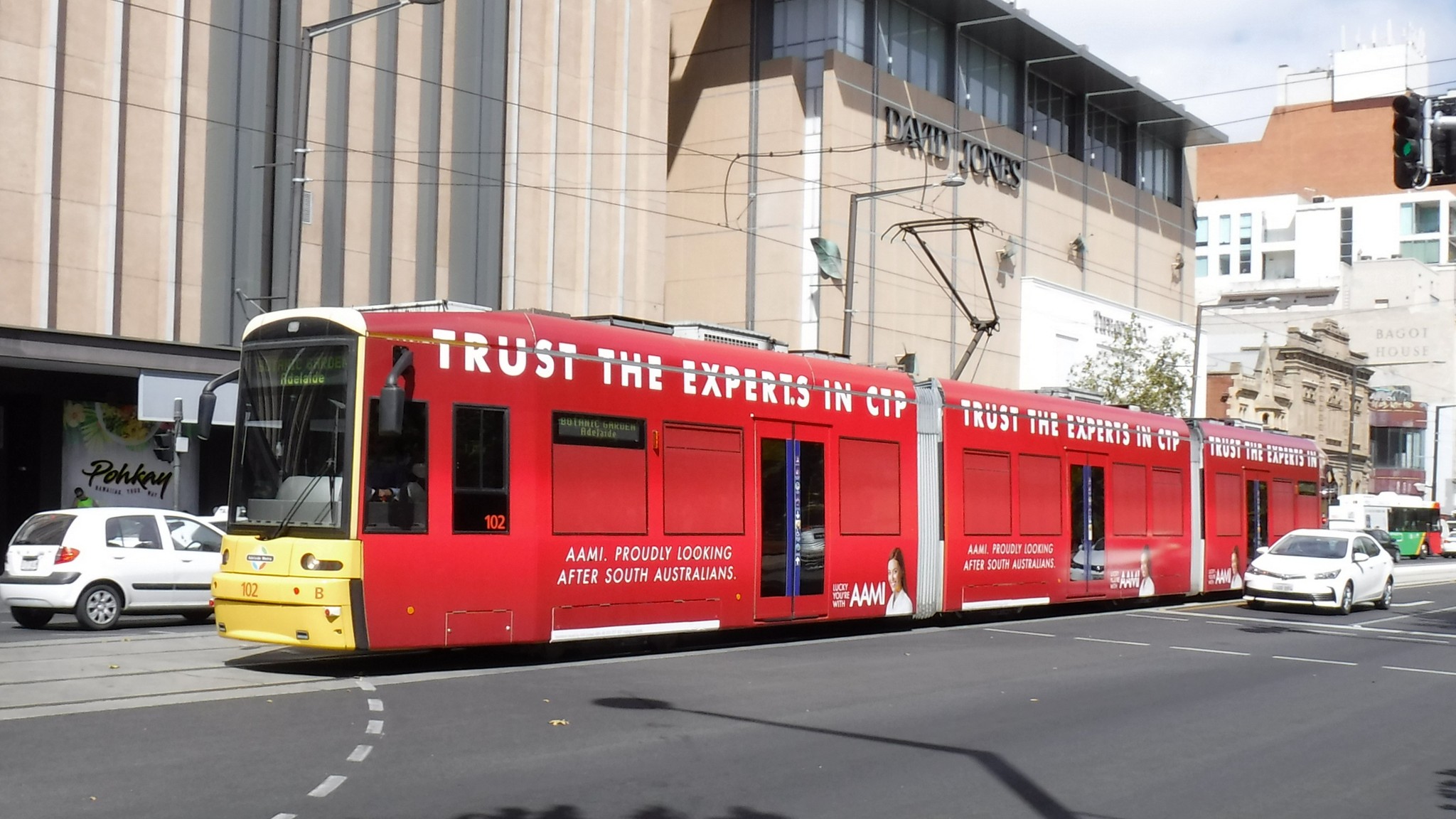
[370,427,425,503]
[1138,545,1154,597]
[1230,547,1243,589]
[885,547,913,615]
[71,488,99,508]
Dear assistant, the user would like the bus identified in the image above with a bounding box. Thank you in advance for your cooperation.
[195,298,1337,655]
[1329,490,1444,560]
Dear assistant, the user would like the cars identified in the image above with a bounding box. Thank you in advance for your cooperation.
[1441,520,1456,558]
[107,503,249,554]
[1241,528,1396,616]
[0,506,226,631]
